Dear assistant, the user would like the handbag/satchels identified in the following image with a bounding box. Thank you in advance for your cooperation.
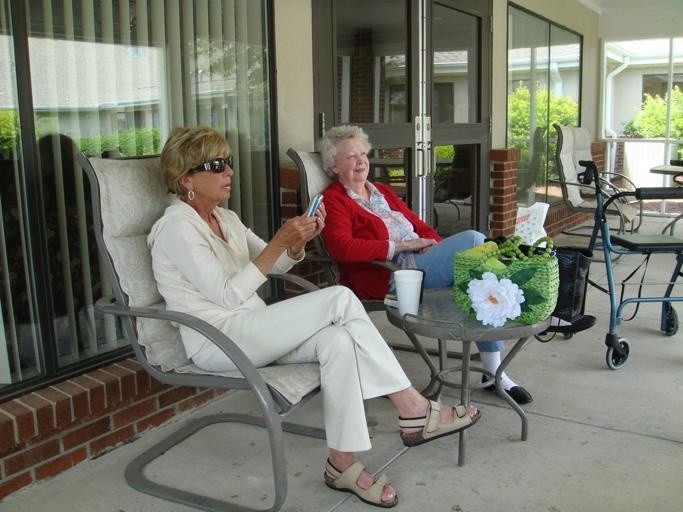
[453,235,560,329]
[484,238,593,320]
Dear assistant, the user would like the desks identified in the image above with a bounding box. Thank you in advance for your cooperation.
[368,157,452,179]
[384,286,553,468]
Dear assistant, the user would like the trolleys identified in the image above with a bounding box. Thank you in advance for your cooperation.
[555,155,681,373]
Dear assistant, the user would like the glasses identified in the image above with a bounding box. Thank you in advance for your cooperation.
[189,155,234,173]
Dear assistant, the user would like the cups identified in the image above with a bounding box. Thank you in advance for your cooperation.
[394,268,424,317]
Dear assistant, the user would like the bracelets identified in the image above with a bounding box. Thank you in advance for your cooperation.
[288,247,304,258]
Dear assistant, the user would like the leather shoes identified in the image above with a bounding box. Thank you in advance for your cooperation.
[538,315,596,335]
[482,373,533,404]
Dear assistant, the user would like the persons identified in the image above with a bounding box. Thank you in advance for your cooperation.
[308,120,536,407]
[142,121,486,509]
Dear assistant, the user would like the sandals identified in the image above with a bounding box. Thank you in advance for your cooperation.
[398,399,481,447]
[324,457,398,508]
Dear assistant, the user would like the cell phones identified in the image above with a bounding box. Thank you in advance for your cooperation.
[307,193,323,218]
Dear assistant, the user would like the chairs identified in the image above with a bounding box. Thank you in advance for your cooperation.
[286,147,481,398]
[517,125,550,209]
[72,150,321,512]
[432,144,476,227]
[551,122,644,263]
